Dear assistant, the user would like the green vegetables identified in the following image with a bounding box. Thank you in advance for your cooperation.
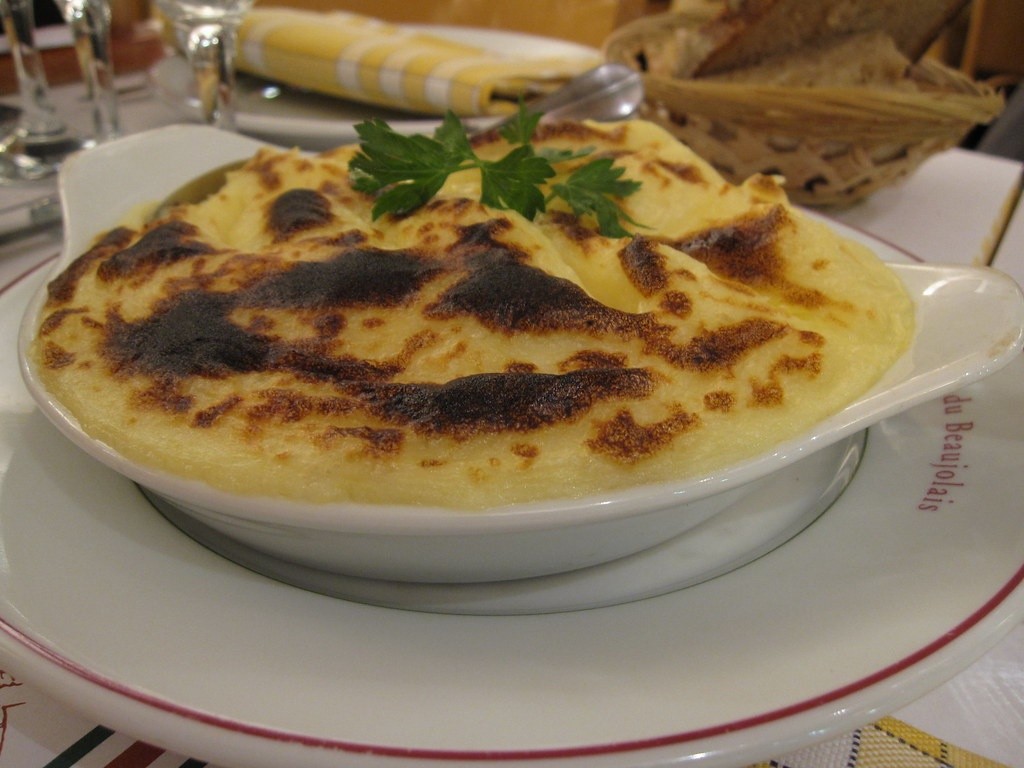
[344,97,654,243]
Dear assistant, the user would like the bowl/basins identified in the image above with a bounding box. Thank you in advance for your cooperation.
[16,123,1024,582]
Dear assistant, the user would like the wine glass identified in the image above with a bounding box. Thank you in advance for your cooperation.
[156,0,255,131]
[54,0,124,143]
[0,0,97,170]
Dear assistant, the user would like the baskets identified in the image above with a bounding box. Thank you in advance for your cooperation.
[604,13,1004,211]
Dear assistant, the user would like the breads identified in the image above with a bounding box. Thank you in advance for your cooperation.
[654,0,971,94]
[23,111,915,511]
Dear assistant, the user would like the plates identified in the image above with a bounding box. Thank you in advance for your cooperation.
[149,24,611,148]
[0,203,1024,768]
